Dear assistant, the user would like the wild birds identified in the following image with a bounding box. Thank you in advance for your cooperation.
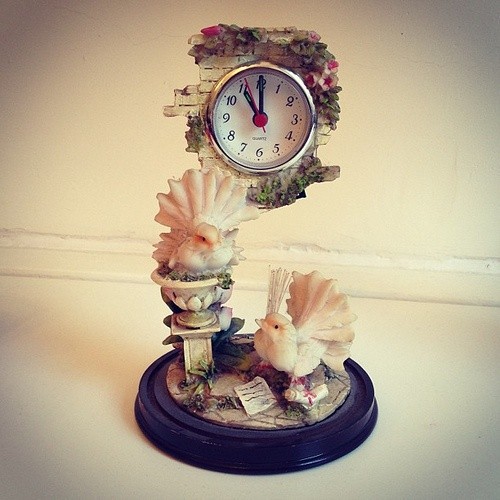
[255,270,358,381]
[151,165,261,271]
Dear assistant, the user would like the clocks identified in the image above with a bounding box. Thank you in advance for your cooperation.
[205,59,317,175]
